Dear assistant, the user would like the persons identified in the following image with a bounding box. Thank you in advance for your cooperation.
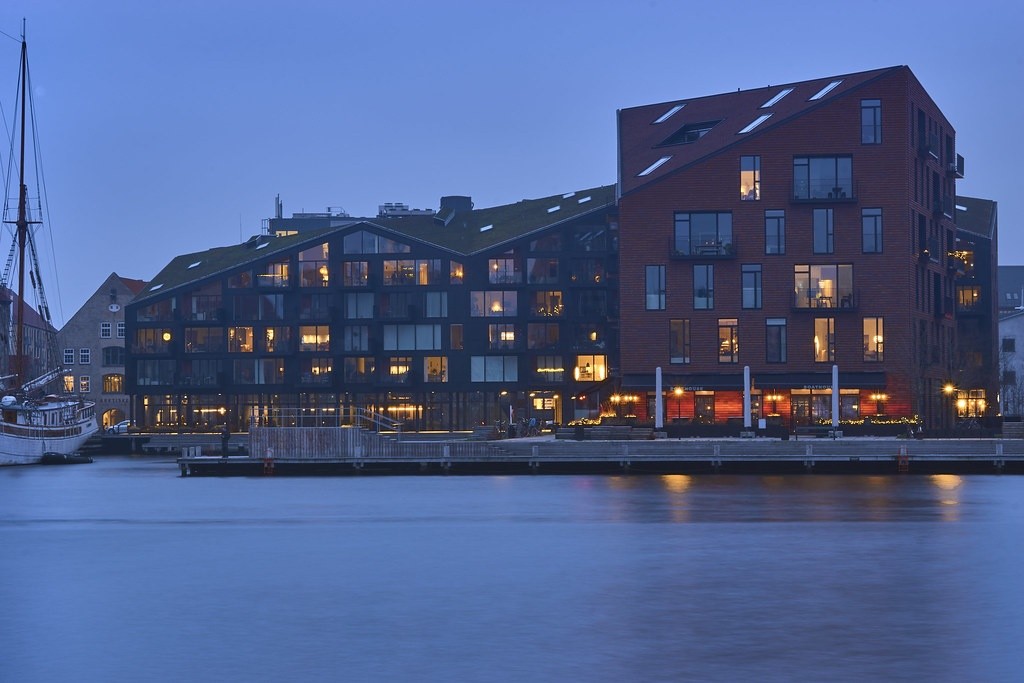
[433,370,445,377]
[508,416,543,437]
[391,266,406,284]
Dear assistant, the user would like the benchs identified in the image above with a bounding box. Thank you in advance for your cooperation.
[795,426,836,440]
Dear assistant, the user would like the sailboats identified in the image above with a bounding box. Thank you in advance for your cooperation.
[0,16,97,465]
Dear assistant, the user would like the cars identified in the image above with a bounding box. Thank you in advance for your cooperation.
[107,419,130,434]
